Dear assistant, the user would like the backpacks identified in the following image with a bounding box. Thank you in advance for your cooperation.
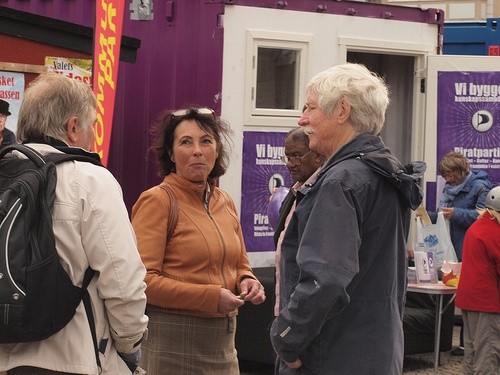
[0,143,105,344]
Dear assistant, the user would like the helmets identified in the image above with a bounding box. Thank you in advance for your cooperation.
[484,186,500,211]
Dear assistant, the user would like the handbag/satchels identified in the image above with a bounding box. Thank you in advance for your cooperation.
[415,210,459,280]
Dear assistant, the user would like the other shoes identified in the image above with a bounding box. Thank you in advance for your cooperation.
[451,346,464,355]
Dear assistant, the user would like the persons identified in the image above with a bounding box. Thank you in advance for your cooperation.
[414,153,494,357]
[0,69,150,375]
[129,104,267,375]
[272,126,327,252]
[268,62,427,375]
[455,185,500,375]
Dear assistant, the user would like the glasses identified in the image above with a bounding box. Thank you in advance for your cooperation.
[170,108,216,119]
[280,150,312,166]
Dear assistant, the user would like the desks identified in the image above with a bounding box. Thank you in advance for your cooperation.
[407,281,457,375]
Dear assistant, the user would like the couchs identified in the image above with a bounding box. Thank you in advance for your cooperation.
[234,268,455,375]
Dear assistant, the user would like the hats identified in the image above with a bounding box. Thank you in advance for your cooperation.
[0,99,11,116]
